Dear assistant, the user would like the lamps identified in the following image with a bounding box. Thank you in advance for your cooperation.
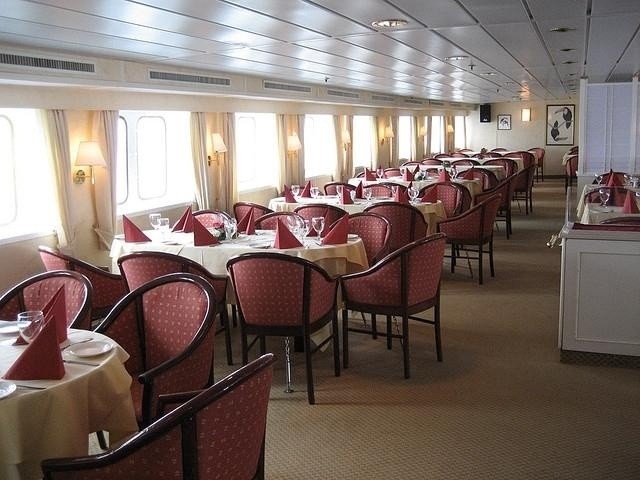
[521,108,531,122]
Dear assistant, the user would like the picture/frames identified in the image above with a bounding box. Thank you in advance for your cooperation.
[546,104,575,145]
[497,114,511,130]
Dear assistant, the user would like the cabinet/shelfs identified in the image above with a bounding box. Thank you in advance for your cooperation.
[557,223,640,369]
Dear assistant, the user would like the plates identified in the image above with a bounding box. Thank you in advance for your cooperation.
[347,234,358,240]
[70,341,113,357]
[0,381,18,399]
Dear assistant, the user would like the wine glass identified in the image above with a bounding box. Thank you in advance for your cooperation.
[288,183,419,206]
[599,188,610,209]
[142,212,326,248]
[372,167,459,181]
[594,173,604,187]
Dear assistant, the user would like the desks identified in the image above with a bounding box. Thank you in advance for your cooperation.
[580,203,640,224]
[576,184,640,210]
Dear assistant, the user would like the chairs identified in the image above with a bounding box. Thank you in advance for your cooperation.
[592,172,628,184]
[562,146,578,195]
[584,188,640,206]
[599,216,640,225]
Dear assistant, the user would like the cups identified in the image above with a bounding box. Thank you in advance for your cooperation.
[17,311,45,343]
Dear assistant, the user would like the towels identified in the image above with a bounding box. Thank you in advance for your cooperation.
[606,172,624,186]
[623,190,640,213]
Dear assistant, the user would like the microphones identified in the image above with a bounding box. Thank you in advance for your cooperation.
[479,104,491,122]
